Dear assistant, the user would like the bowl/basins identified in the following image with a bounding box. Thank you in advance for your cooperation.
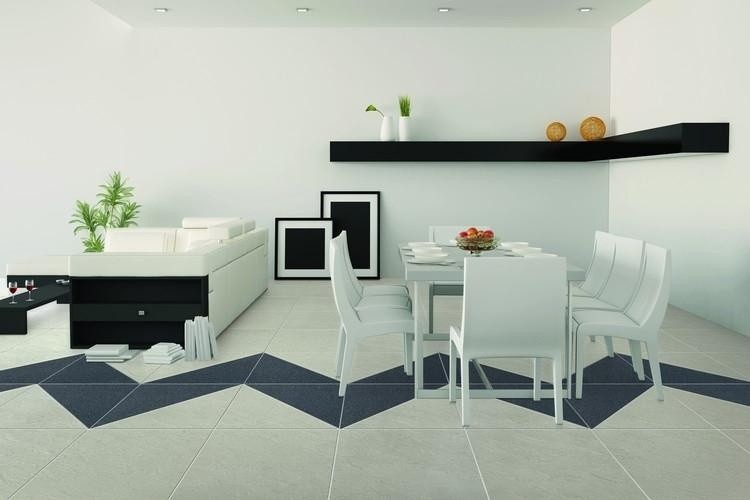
[411,246,443,254]
[414,252,449,263]
[512,247,542,256]
[500,240,529,249]
[407,241,435,250]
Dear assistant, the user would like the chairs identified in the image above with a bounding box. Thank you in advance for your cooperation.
[566,229,619,343]
[574,242,672,402]
[341,229,409,297]
[428,225,487,240]
[449,255,568,429]
[338,234,412,372]
[568,238,646,387]
[329,239,414,398]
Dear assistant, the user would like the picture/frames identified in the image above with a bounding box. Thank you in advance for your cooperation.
[274,217,334,281]
[321,190,380,281]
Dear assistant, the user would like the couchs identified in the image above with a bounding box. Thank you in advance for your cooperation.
[70,218,268,349]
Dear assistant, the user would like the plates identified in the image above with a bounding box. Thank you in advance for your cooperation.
[404,251,415,257]
[496,245,508,250]
[405,257,457,266]
[503,250,518,256]
[401,246,412,251]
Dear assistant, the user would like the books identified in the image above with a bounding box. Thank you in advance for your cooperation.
[142,315,219,364]
[84,343,135,363]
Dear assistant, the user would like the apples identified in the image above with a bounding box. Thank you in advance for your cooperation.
[467,233,477,238]
[467,228,477,235]
[459,231,468,238]
[478,231,484,238]
[483,231,494,238]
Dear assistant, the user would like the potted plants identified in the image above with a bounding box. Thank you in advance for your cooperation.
[365,105,393,141]
[397,94,412,141]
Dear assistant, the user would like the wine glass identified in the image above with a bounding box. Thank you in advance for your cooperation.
[8,281,19,305]
[24,278,35,302]
